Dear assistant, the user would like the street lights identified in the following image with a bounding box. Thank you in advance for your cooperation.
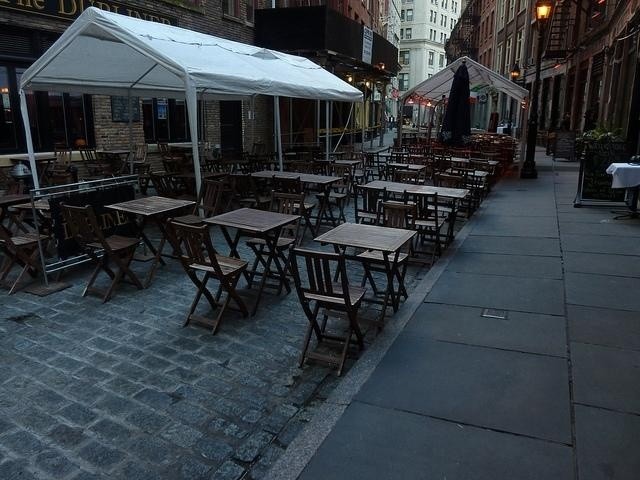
[517,1,556,180]
[507,61,522,135]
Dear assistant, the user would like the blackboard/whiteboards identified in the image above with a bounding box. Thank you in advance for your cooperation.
[580,143,627,202]
[554,130,574,158]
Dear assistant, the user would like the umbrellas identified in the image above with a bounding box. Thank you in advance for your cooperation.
[441,60,471,146]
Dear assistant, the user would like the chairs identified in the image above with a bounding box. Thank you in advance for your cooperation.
[1,128,518,297]
[167,218,249,337]
[60,202,145,304]
[289,244,368,377]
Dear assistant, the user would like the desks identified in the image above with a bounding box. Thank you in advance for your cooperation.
[204,208,302,318]
[603,158,638,222]
[314,223,417,337]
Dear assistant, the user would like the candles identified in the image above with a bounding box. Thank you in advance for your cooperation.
[575,123,629,154]
